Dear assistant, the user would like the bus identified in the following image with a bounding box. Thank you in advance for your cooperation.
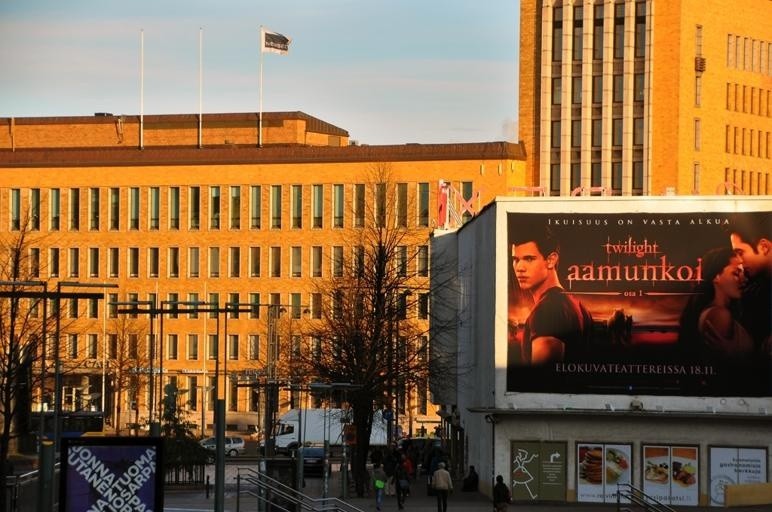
[19,411,105,459]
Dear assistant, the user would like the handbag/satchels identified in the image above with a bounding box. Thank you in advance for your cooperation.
[375,479,385,489]
[398,479,410,490]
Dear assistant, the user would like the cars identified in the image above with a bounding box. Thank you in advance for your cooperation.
[197,447,217,464]
[294,445,335,477]
[397,437,451,472]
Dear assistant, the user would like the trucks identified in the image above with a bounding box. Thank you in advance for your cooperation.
[259,408,388,457]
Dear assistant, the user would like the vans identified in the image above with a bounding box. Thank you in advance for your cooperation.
[198,434,247,458]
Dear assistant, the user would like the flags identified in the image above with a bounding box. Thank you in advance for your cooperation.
[261,31,290,57]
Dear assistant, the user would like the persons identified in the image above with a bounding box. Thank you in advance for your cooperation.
[683,213,772,366]
[350,440,511,512]
[513,225,587,366]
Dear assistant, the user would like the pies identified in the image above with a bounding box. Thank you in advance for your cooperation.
[583,451,602,484]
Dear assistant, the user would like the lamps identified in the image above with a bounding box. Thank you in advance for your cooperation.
[483,413,497,424]
